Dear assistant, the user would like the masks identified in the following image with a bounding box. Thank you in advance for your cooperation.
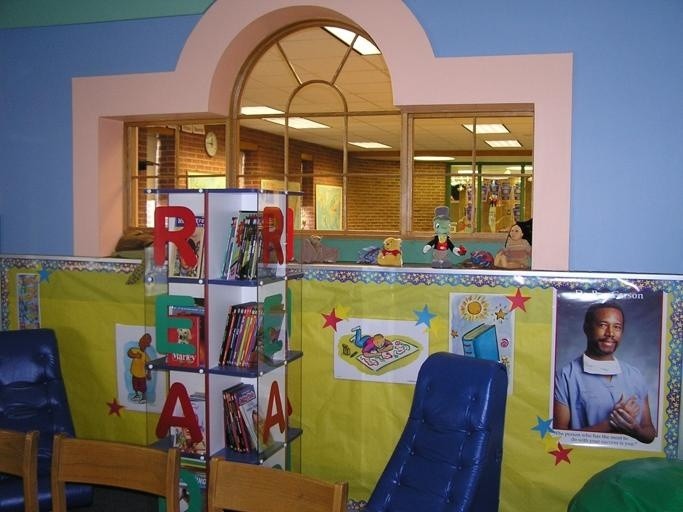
[582,354,622,375]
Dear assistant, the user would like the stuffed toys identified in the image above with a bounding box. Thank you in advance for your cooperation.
[311,235,324,259]
[422,205,468,268]
[377,237,404,267]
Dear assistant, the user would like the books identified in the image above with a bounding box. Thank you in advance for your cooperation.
[172,215,205,279]
[220,210,278,280]
[166,305,205,369]
[174,390,206,462]
[221,382,275,454]
[219,301,285,368]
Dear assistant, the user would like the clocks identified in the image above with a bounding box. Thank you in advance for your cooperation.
[204,131,218,158]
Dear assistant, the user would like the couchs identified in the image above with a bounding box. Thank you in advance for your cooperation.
[1,329,95,511]
[351,350,509,512]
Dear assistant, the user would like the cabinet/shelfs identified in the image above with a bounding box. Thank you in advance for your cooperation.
[142,182,304,512]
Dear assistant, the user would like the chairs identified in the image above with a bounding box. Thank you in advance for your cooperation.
[50,431,182,512]
[207,455,349,512]
[1,428,41,511]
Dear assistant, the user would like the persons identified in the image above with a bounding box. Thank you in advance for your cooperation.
[127,333,153,404]
[553,302,656,444]
[494,218,532,269]
[349,325,394,358]
